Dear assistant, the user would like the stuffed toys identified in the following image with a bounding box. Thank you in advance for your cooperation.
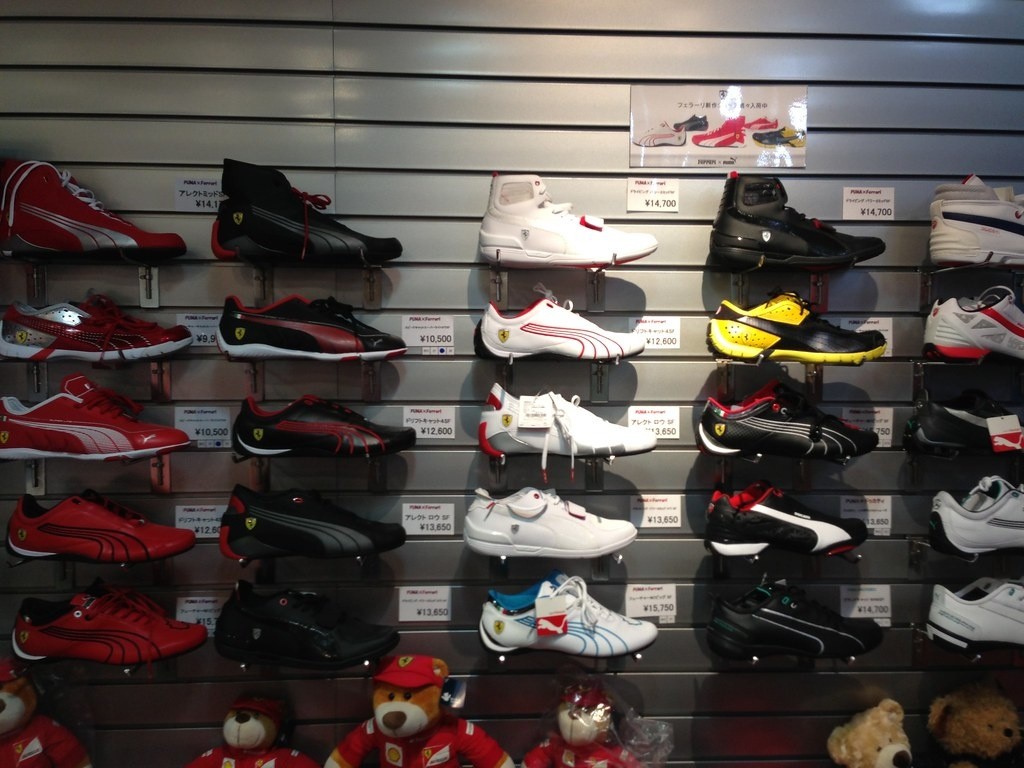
[927,678,1024,768]
[324,655,515,768]
[186,683,322,768]
[0,657,93,768]
[519,681,639,768]
[828,699,914,768]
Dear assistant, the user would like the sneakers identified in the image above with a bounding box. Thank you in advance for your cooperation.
[463,487,638,560]
[219,483,406,559]
[902,389,1024,457]
[634,114,807,147]
[705,286,887,364]
[930,174,1024,264]
[697,380,880,460]
[0,157,189,264]
[477,383,657,483]
[478,568,658,658]
[929,475,1024,556]
[704,480,868,556]
[213,578,401,670]
[921,285,1024,362]
[706,579,884,659]
[926,577,1024,650]
[709,170,886,273]
[6,489,208,667]
[211,158,403,264]
[0,372,191,461]
[231,393,416,460]
[478,172,659,269]
[0,293,193,362]
[473,281,645,362]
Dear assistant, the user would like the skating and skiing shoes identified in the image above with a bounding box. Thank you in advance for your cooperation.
[216,294,408,362]
